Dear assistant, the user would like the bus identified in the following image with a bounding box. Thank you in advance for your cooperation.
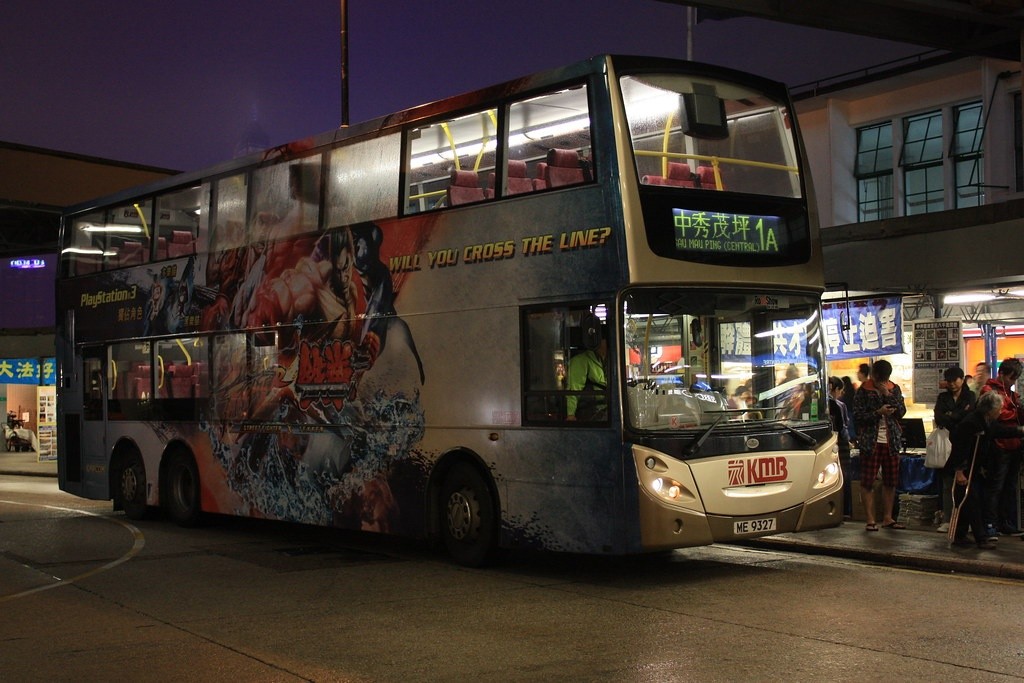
[55,55,851,565]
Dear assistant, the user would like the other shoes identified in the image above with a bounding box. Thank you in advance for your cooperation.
[843,514,852,521]
[978,540,996,549]
[937,523,951,533]
[953,535,974,545]
[987,534,999,542]
[1011,529,1024,536]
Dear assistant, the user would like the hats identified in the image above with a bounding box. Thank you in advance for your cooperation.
[943,367,964,381]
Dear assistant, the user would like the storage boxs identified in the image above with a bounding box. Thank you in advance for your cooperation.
[852,480,884,522]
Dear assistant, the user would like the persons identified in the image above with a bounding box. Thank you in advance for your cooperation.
[735,377,752,403]
[777,364,800,385]
[855,360,907,531]
[934,357,1024,553]
[827,376,856,520]
[12,422,21,430]
[566,323,607,421]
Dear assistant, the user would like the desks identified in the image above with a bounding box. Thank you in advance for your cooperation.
[849,449,941,518]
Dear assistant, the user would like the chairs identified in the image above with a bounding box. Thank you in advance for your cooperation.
[560,326,585,358]
[76,191,398,272]
[445,146,723,207]
[112,362,203,399]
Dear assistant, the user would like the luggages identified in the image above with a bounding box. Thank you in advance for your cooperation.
[898,455,937,495]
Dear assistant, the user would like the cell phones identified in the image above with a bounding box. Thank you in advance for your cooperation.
[886,405,897,408]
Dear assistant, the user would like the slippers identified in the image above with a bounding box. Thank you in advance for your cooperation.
[865,523,879,532]
[881,521,907,530]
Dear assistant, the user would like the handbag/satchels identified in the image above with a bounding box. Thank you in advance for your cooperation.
[923,425,952,468]
